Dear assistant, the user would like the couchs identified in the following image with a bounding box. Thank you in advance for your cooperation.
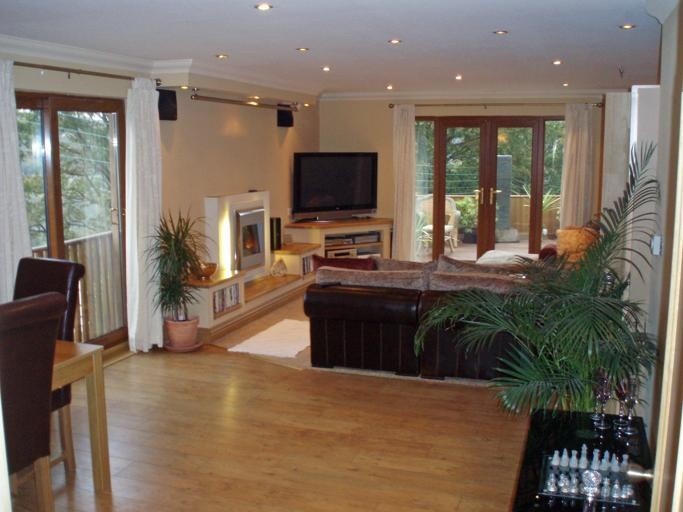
[303,225,597,380]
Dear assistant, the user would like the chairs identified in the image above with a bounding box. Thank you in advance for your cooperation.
[413,193,462,247]
[0,257,113,512]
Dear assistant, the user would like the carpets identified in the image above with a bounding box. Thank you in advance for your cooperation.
[210,293,313,371]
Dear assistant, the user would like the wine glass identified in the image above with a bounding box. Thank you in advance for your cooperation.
[590,365,641,438]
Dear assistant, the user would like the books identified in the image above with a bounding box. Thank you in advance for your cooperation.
[323,231,382,254]
[213,284,239,313]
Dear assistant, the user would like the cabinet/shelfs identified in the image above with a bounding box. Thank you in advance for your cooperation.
[181,189,395,343]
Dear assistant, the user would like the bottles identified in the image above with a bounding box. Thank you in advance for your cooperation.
[544,443,638,507]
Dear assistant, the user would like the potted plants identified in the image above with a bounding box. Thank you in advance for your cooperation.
[142,207,217,355]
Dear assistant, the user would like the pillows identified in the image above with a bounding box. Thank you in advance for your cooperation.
[556,228,597,262]
[311,254,525,272]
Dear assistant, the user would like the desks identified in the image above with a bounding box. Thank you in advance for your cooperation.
[511,409,649,512]
[417,234,455,254]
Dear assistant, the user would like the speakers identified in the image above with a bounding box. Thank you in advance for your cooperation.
[271,217,281,251]
[156,89,178,120]
[277,105,293,127]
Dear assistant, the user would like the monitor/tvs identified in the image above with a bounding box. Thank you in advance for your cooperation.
[292,151,379,223]
